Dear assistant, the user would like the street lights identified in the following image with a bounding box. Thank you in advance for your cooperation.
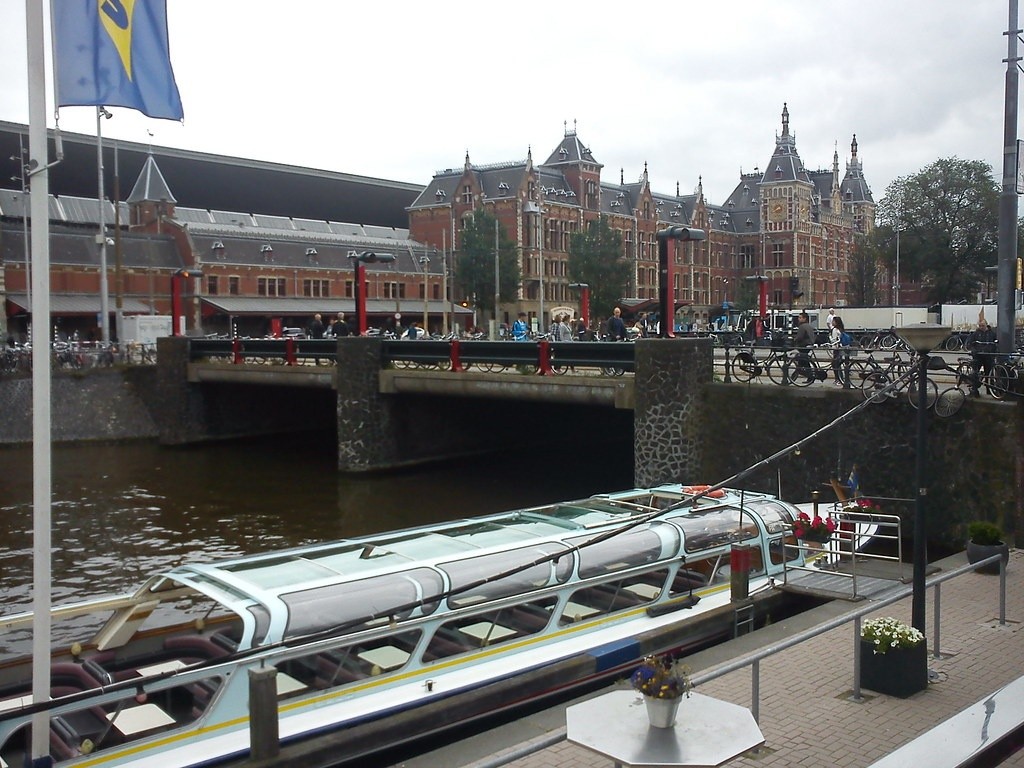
[346,250,395,336]
[655,225,705,337]
[170,267,204,336]
[747,276,768,318]
[569,282,588,328]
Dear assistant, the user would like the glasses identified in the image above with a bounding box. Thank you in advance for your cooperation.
[798,316,805,319]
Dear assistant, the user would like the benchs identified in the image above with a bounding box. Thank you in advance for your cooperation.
[39,523,785,759]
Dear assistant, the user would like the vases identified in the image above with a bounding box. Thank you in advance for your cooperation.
[636,691,683,730]
[861,639,930,701]
[804,539,824,557]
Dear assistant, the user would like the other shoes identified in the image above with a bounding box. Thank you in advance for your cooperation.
[802,381,814,383]
[572,370,579,374]
[556,369,563,373]
[833,380,842,385]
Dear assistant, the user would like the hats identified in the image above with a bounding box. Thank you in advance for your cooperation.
[518,312,529,317]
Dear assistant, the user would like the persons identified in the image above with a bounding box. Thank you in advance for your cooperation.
[828,317,843,385]
[331,312,348,337]
[640,314,649,338]
[578,318,586,331]
[511,312,529,341]
[560,315,578,373]
[552,315,562,372]
[309,314,325,365]
[793,313,813,384]
[970,319,996,397]
[827,308,836,333]
[405,322,417,340]
[327,318,335,334]
[763,313,770,330]
[607,308,626,342]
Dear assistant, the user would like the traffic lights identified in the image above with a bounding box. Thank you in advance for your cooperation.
[462,301,468,309]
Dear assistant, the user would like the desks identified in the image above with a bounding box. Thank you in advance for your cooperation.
[132,659,187,681]
[622,583,673,600]
[265,670,309,696]
[566,689,766,767]
[104,702,176,736]
[0,691,51,710]
[358,645,411,671]
[546,599,600,619]
[459,622,517,643]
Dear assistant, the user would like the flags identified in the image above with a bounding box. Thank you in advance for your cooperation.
[846,471,859,492]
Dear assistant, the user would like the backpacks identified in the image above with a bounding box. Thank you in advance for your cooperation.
[831,327,851,347]
[511,321,526,338]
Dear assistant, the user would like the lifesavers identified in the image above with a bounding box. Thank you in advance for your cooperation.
[681,485,726,497]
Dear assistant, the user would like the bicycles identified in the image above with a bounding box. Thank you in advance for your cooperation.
[206,332,626,376]
[945,325,976,352]
[935,355,1018,418]
[732,325,938,410]
[2,340,158,373]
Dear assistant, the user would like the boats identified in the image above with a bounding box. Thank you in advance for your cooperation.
[0,483,878,768]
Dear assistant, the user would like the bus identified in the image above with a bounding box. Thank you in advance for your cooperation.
[737,309,820,339]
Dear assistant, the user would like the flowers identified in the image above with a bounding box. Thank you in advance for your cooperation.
[625,653,698,702]
[861,616,921,656]
[838,499,886,519]
[792,512,835,539]
